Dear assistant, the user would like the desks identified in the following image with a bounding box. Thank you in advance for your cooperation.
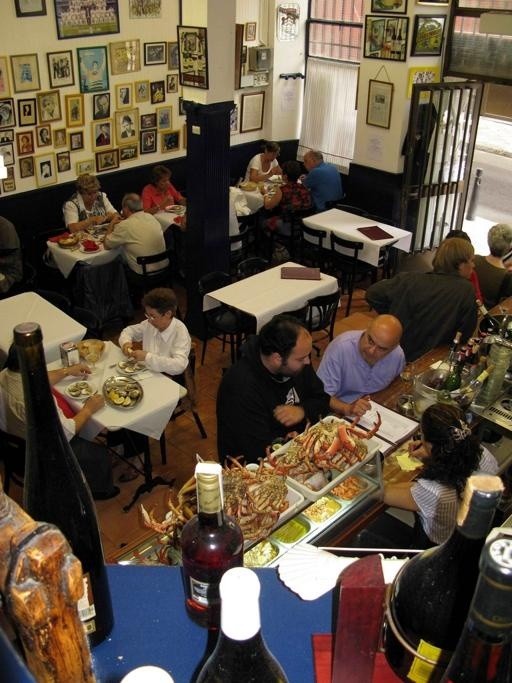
[202,260,340,363]
[302,208,414,312]
[33,339,189,514]
[0,290,89,360]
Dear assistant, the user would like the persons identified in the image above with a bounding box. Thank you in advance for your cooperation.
[64,173,117,229]
[365,220,511,359]
[176,195,244,283]
[301,149,345,212]
[255,159,312,261]
[314,314,411,420]
[0,337,122,501]
[100,287,193,483]
[103,193,173,324]
[382,401,501,552]
[242,142,285,186]
[1,51,177,193]
[217,314,327,468]
[1,212,24,295]
[142,164,188,214]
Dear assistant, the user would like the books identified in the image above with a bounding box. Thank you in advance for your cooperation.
[358,226,392,239]
[281,264,321,280]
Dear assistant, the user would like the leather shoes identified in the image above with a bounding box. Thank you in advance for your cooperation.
[119,463,143,480]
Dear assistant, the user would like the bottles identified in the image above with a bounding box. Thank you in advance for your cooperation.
[383,23,404,58]
[180,459,245,628]
[12,323,118,656]
[430,330,495,390]
[379,469,511,683]
[120,664,175,683]
[195,568,290,682]
[475,298,511,342]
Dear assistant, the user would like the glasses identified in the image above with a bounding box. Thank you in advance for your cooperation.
[144,312,163,320]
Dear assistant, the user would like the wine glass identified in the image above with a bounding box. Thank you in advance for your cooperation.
[86,350,100,370]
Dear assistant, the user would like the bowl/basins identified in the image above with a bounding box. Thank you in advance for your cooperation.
[78,338,105,357]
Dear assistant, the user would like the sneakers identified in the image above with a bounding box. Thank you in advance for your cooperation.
[272,246,290,261]
[92,485,120,499]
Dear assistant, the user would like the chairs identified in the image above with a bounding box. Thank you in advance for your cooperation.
[42,161,344,320]
[38,290,103,340]
[297,220,376,316]
[196,256,272,367]
[157,343,207,467]
[2,430,27,496]
[277,287,340,359]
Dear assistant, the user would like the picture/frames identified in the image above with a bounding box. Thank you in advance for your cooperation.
[362,13,410,64]
[243,22,259,43]
[369,0,410,15]
[176,23,211,91]
[0,0,188,193]
[365,78,395,130]
[237,89,266,135]
[406,64,442,102]
[409,13,449,59]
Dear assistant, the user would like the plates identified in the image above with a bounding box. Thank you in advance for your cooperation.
[58,227,105,253]
[116,360,147,374]
[102,375,144,408]
[66,381,98,399]
[264,172,283,196]
[165,204,186,212]
